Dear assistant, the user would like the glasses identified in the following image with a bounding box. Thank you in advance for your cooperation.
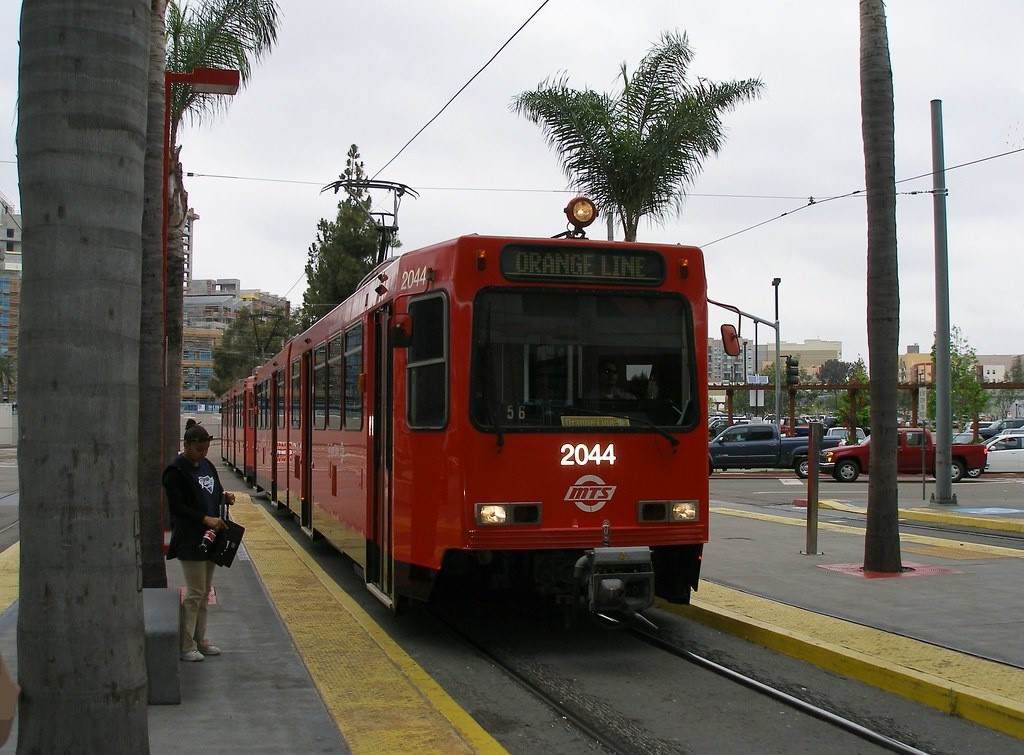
[191,436,214,443]
[598,369,617,375]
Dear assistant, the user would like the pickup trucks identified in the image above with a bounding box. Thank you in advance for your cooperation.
[708,424,841,479]
[819,427,987,482]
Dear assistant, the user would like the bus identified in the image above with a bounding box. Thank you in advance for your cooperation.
[218,196,710,621]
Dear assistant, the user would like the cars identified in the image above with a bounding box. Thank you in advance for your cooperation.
[980,433,1024,473]
[706,408,1024,445]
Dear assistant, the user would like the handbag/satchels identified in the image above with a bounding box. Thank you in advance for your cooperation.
[208,492,245,568]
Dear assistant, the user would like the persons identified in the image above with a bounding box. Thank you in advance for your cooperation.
[163,419,235,663]
[584,360,637,399]
[1006,438,1016,449]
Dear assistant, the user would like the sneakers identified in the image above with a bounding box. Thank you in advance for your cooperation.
[198,645,221,656]
[180,650,204,662]
